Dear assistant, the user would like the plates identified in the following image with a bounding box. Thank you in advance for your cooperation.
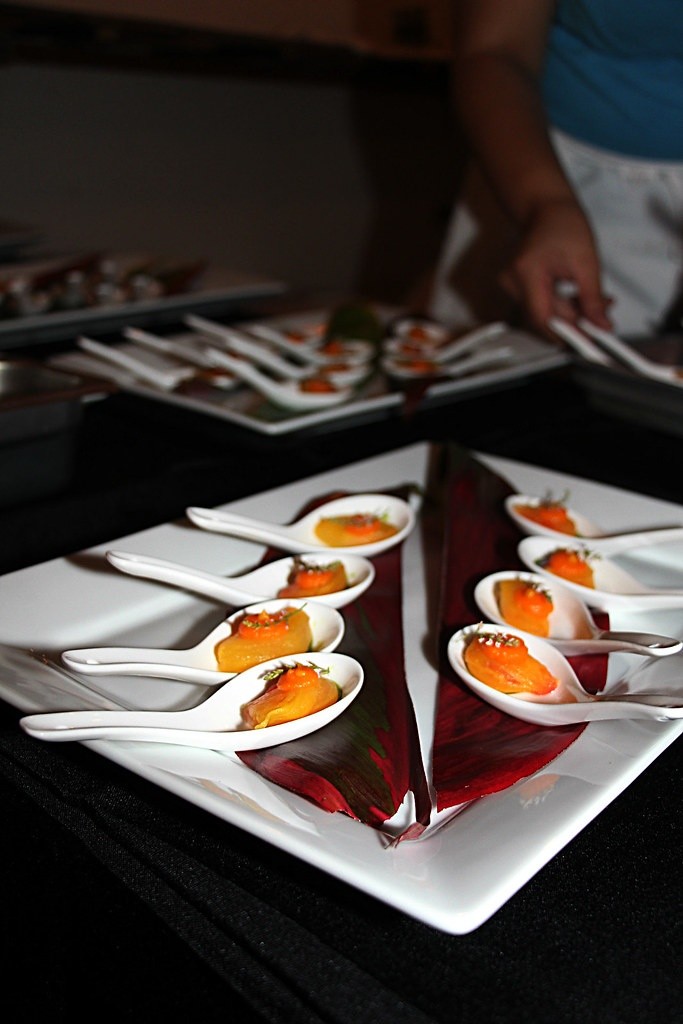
[48,312,572,436]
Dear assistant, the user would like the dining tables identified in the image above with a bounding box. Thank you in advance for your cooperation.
[0,311,682,1024]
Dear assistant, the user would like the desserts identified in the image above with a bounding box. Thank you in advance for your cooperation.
[214,489,596,728]
[281,320,438,394]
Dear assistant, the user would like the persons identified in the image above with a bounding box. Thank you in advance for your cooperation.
[428,0,683,356]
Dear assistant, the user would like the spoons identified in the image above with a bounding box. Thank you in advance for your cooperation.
[187,493,415,557]
[474,571,683,657]
[61,599,345,687]
[79,315,374,411]
[579,319,683,387]
[0,443,683,935]
[448,624,683,726]
[384,320,512,378]
[507,495,683,553]
[106,552,376,609]
[19,652,364,751]
[519,536,683,613]
